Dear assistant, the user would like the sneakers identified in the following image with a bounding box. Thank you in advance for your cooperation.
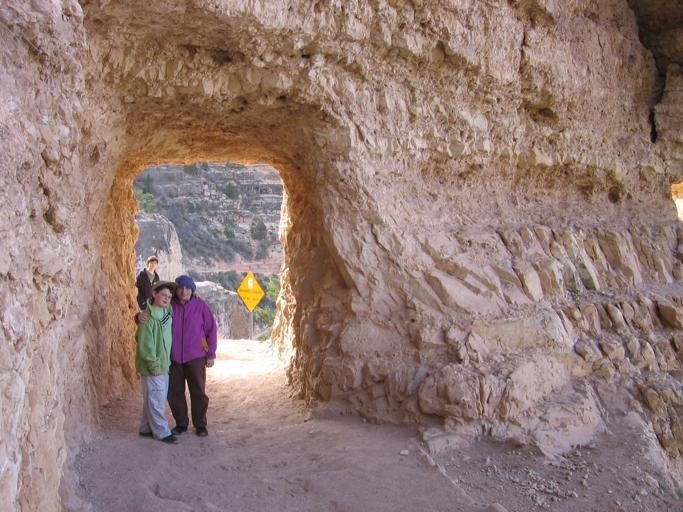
[195,426,208,436]
[171,425,187,434]
[140,432,177,442]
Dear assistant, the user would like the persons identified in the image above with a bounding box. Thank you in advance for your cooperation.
[135,256,160,310]
[135,275,217,436]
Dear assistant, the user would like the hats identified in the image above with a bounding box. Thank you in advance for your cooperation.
[175,275,196,292]
[152,280,177,291]
